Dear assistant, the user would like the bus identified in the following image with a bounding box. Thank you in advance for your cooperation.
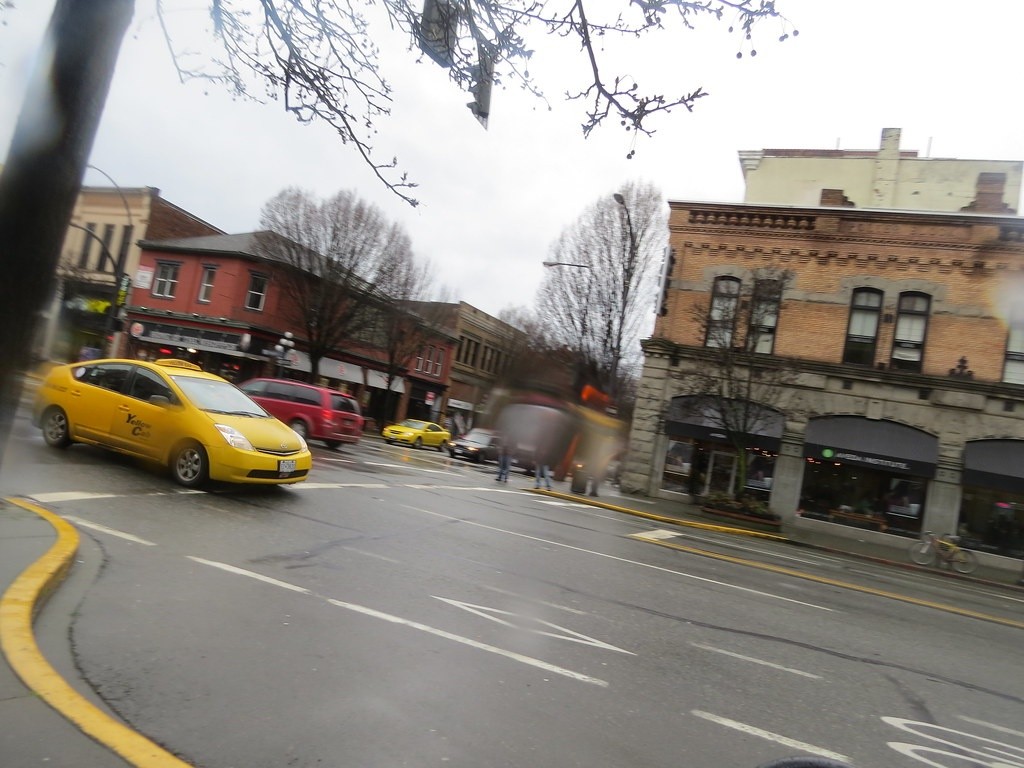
[498,394,629,479]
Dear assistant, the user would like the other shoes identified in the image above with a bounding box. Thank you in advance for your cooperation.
[534,486,539,489]
[504,478,509,482]
[495,478,502,481]
[546,486,551,490]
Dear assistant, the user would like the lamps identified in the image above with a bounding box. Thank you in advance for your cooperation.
[884,314,893,323]
[669,354,679,366]
[220,318,227,321]
[1003,399,1017,413]
[919,388,931,401]
[661,307,668,317]
[142,306,150,312]
[842,379,854,389]
[192,312,200,317]
[878,362,886,368]
[753,366,764,378]
[166,309,173,314]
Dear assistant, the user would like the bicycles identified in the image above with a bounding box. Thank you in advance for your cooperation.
[908,530,978,575]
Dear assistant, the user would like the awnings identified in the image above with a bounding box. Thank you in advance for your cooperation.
[802,413,940,482]
[664,393,783,457]
[962,438,1024,500]
[137,336,269,362]
[280,348,404,394]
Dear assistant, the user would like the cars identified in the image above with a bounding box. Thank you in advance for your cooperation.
[30,357,312,491]
[381,419,452,452]
[446,427,501,464]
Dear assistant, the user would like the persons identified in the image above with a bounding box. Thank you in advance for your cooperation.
[531,438,552,491]
[495,434,511,483]
[442,410,454,438]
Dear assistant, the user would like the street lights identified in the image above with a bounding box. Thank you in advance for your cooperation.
[272,331,295,379]
[604,192,636,411]
[542,261,594,385]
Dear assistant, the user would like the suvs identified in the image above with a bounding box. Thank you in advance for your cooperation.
[235,377,365,451]
